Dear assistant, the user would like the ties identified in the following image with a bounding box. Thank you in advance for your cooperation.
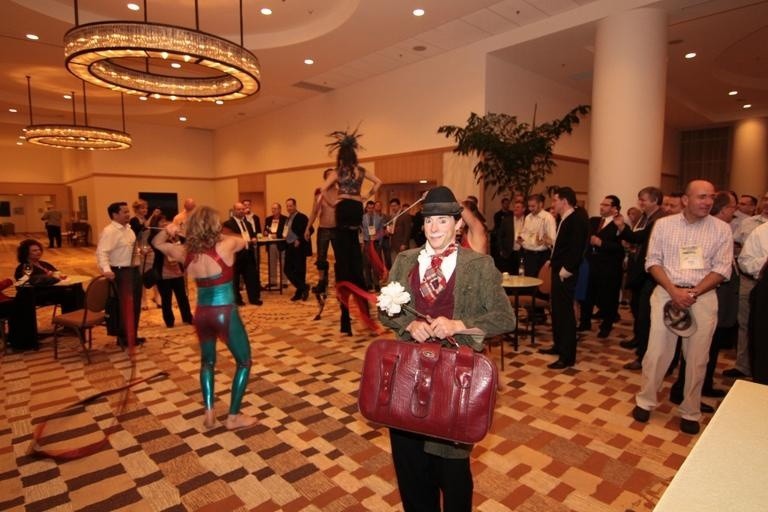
[635,212,647,231]
[418,243,456,305]
[598,218,605,233]
[240,220,246,233]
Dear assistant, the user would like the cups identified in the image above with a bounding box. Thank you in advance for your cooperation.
[257,224,273,240]
[503,272,509,280]
[142,239,151,253]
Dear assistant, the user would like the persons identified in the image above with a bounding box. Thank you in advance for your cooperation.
[304,168,344,292]
[320,141,382,284]
[0,278,42,352]
[632,180,735,435]
[374,185,519,511]
[668,190,739,413]
[151,204,257,431]
[96,178,767,385]
[41,204,63,248]
[14,239,86,335]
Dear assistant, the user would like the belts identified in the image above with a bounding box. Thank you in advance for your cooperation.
[110,266,138,271]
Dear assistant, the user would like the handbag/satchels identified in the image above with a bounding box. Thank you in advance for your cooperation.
[357,328,501,446]
[142,262,164,290]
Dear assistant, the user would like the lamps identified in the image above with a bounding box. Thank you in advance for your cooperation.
[63,0,261,102]
[26,75,133,151]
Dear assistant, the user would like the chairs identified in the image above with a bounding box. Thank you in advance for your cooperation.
[0,278,16,302]
[53,274,128,364]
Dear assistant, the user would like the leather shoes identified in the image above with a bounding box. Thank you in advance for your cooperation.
[290,294,302,302]
[623,359,643,370]
[538,346,559,355]
[668,392,714,413]
[722,368,747,378]
[546,359,575,369]
[237,300,246,306]
[249,299,263,306]
[619,338,637,350]
[302,284,311,301]
[702,386,727,398]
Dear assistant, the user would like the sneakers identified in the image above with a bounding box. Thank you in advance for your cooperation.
[596,327,610,341]
[152,297,163,308]
[312,281,327,294]
[592,311,604,320]
[137,337,146,343]
[632,405,650,423]
[142,305,148,310]
[575,322,592,331]
[680,416,700,434]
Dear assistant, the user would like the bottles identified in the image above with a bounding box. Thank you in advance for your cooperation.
[387,223,390,232]
[538,218,546,241]
[519,258,525,277]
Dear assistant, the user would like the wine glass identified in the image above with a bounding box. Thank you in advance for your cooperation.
[23,263,33,284]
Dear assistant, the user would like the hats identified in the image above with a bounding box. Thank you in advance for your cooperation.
[662,299,698,339]
[420,186,464,217]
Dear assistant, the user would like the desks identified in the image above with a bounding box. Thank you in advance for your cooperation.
[258,239,288,295]
[501,276,544,351]
[0,283,86,353]
